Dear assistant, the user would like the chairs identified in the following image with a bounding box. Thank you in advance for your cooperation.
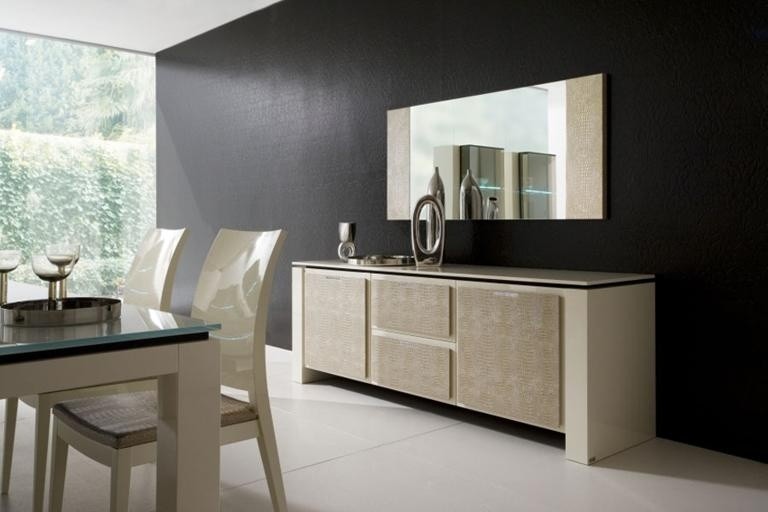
[0,225,189,512]
[47,225,289,512]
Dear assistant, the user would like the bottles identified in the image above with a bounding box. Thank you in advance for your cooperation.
[425,166,446,252]
[486,197,498,219]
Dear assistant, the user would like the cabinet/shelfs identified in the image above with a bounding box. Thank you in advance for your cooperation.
[456,279,564,434]
[459,144,504,220]
[303,266,368,384]
[368,272,456,407]
[517,150,557,219]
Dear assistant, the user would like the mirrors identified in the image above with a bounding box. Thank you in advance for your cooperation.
[384,71,610,223]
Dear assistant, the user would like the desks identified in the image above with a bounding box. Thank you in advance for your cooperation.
[0,278,222,512]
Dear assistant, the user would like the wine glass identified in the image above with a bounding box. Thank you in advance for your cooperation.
[0,244,81,311]
